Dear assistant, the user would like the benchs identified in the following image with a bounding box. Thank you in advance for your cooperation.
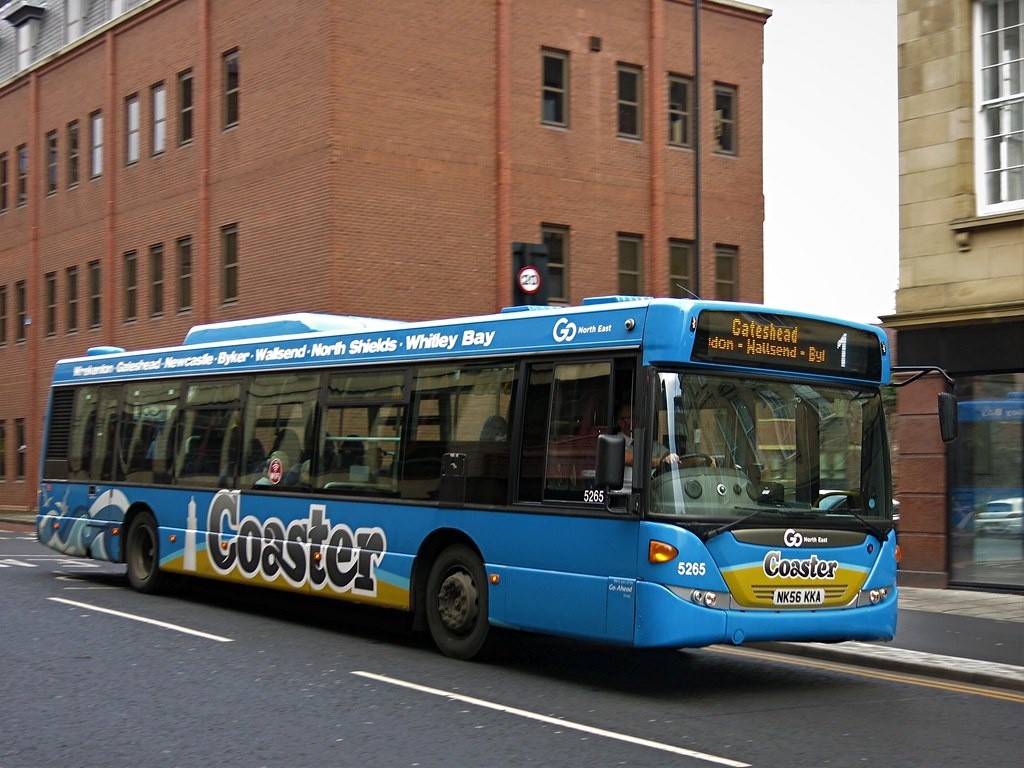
[121,465,393,497]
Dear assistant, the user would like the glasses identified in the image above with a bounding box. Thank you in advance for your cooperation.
[619,415,635,420]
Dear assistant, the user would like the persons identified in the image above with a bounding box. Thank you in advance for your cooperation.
[607,399,717,510]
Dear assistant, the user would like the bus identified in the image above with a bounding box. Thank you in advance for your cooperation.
[37,279,961,668]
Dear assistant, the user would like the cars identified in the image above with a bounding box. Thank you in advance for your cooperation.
[973,495,1024,536]
[812,483,900,532]
